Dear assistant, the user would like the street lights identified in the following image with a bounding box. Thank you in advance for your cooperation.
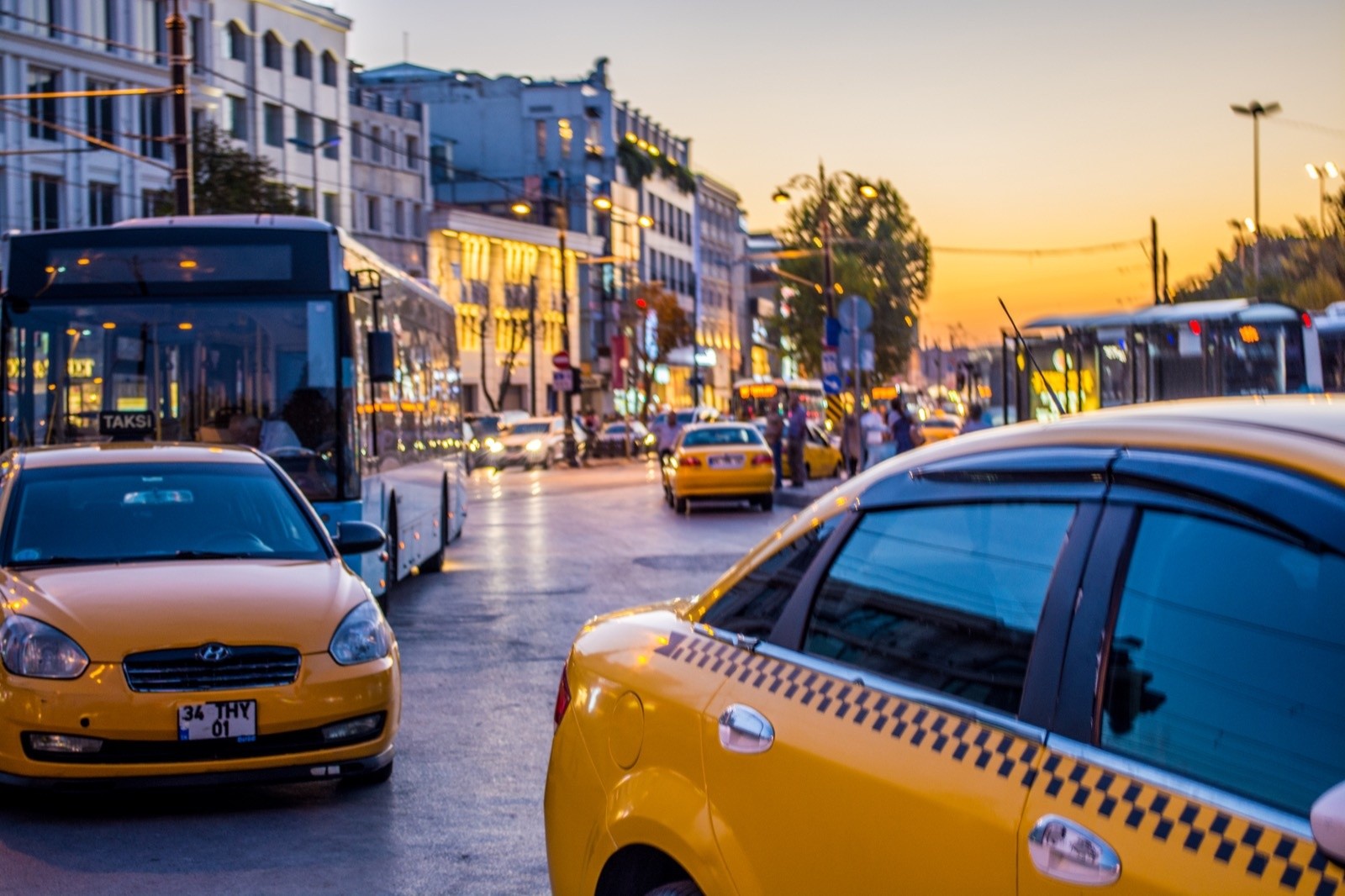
[593,213,654,304]
[773,164,877,316]
[1307,162,1337,226]
[1230,101,1282,271]
[620,358,632,460]
[286,136,343,220]
[513,169,612,468]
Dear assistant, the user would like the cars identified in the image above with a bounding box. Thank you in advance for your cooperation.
[660,422,775,518]
[918,402,965,446]
[464,406,729,469]
[755,419,843,481]
[1,443,401,808]
[544,395,1345,896]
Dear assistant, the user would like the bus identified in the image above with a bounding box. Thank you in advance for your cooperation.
[729,377,828,428]
[0,213,468,615]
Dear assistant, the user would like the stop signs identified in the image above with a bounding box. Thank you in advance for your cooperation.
[553,350,570,369]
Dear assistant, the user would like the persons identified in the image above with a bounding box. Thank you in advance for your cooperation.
[888,398,918,456]
[765,399,784,489]
[959,404,993,435]
[653,410,681,468]
[576,409,656,450]
[786,393,807,489]
[462,416,474,476]
[228,414,302,457]
[840,407,886,476]
